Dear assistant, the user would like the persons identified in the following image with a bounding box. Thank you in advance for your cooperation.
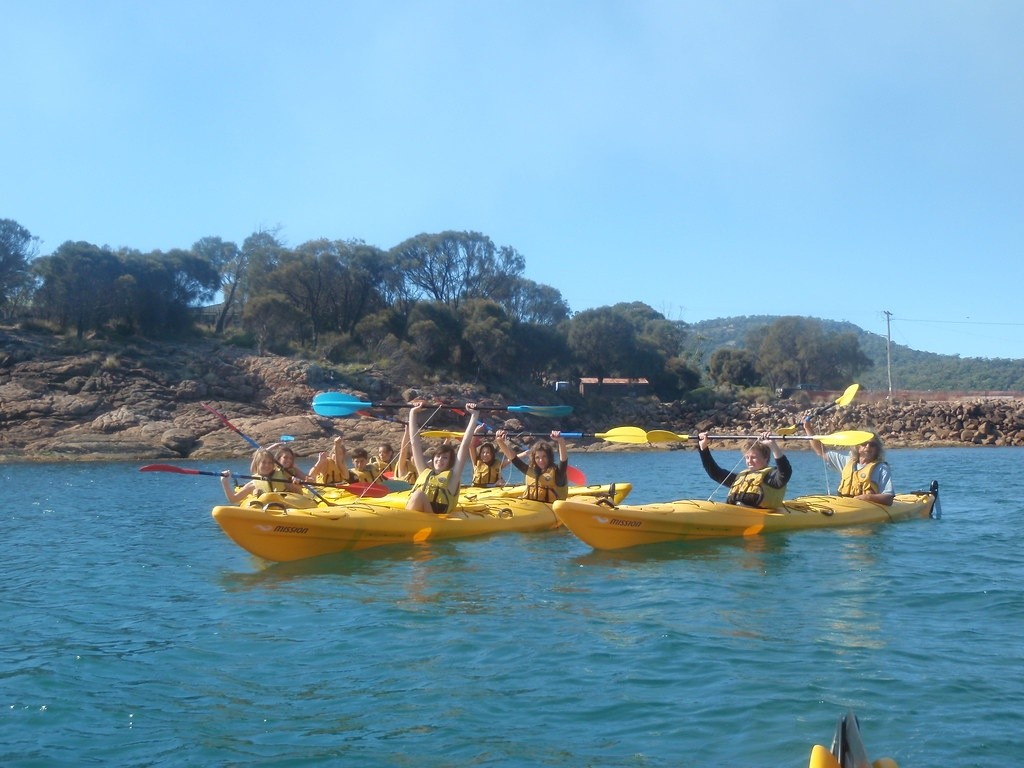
[803,417,896,506]
[219,401,569,516]
[697,431,792,510]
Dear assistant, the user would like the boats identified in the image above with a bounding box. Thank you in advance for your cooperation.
[211,466,633,564]
[551,479,940,554]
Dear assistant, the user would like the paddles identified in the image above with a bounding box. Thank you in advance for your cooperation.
[776,383,863,438]
[199,398,327,504]
[309,392,575,420]
[436,403,589,486]
[418,425,649,446]
[645,427,876,450]
[135,460,389,503]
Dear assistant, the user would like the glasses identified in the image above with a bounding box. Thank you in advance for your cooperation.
[859,441,878,448]
